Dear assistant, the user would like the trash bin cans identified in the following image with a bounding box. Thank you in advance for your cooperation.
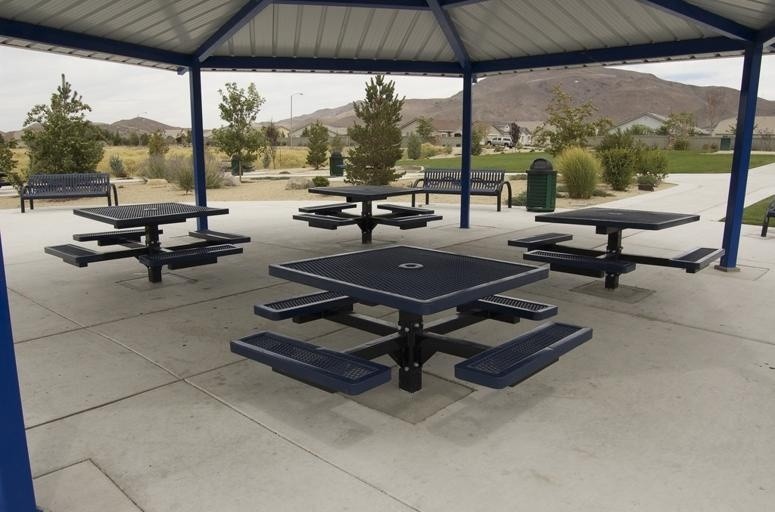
[720,138,730,151]
[330,152,342,176]
[231,155,243,176]
[525,159,557,211]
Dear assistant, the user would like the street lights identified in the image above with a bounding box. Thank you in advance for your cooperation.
[290,92,303,131]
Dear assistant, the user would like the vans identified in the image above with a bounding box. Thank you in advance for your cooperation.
[485,137,514,149]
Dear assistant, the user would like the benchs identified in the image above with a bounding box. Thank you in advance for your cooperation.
[453,294,559,320]
[46,242,105,270]
[21,173,118,213]
[523,249,636,274]
[452,322,594,388]
[189,227,252,245]
[138,244,244,276]
[507,232,574,247]
[74,228,166,244]
[412,168,511,211]
[669,246,726,271]
[251,289,360,321]
[227,330,391,393]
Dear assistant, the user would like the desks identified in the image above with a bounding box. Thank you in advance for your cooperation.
[69,202,230,237]
[310,185,427,245]
[535,207,700,247]
[269,245,550,322]
